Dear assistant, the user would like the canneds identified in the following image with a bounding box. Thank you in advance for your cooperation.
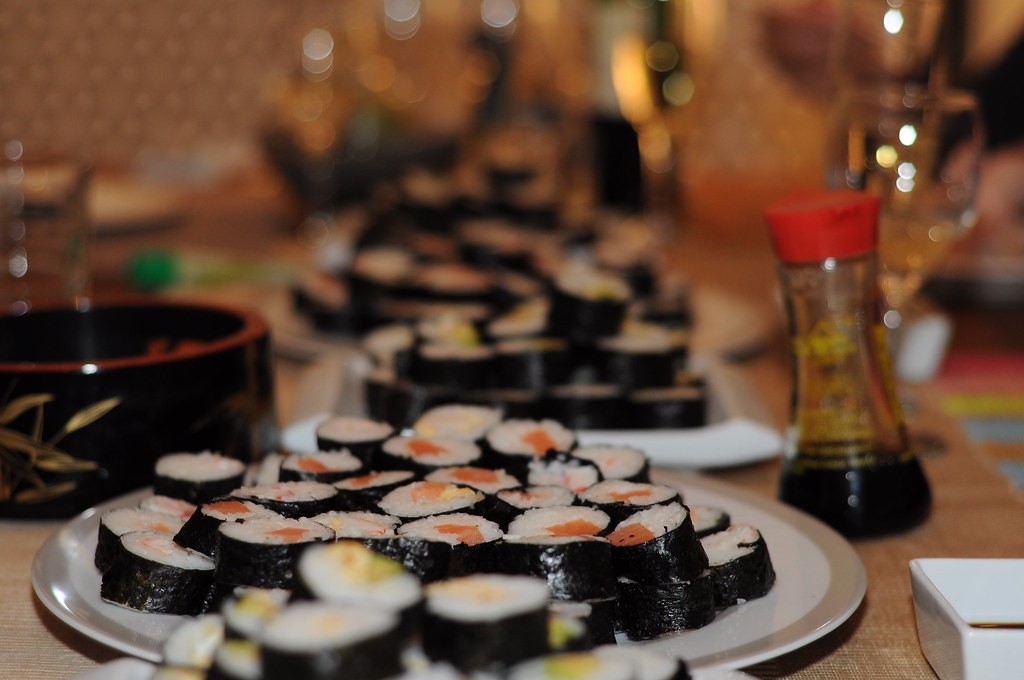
[766,188,934,540]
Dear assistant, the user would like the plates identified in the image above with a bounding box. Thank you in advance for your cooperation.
[30,282,870,680]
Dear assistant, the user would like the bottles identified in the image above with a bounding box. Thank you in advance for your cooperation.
[761,186,939,535]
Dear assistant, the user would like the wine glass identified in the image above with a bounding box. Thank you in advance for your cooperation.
[822,81,989,464]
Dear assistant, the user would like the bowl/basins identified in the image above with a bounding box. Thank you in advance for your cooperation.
[0,298,284,525]
[909,556,1024,680]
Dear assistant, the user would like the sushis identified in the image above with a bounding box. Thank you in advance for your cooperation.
[94,223,776,679]
